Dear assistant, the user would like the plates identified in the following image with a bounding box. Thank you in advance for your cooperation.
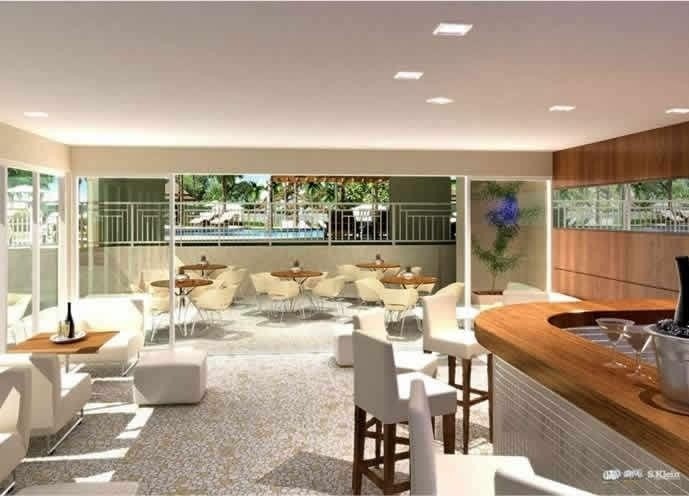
[49,328,87,344]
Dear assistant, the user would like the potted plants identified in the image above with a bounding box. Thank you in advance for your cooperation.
[470,179,543,306]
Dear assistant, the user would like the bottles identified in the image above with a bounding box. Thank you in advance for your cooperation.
[66,302,74,339]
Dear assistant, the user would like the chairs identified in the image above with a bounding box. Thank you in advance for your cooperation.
[0,254,605,496]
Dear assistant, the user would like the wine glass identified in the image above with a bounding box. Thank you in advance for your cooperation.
[595,317,635,369]
[622,325,653,378]
